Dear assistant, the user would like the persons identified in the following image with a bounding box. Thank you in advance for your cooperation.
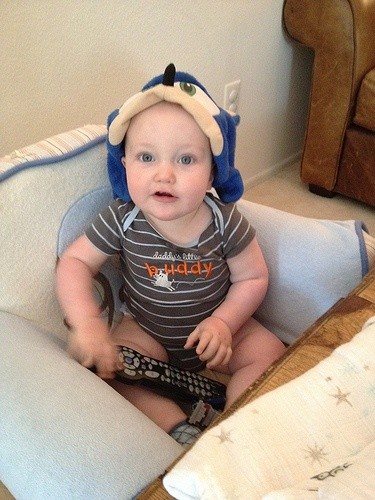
[54,63,286,449]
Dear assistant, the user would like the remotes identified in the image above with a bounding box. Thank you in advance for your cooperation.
[111,344,227,411]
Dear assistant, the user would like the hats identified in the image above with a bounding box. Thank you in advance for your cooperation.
[106,63,244,204]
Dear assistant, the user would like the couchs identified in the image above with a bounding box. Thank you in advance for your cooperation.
[282,1,374,208]
[1,123,374,499]
